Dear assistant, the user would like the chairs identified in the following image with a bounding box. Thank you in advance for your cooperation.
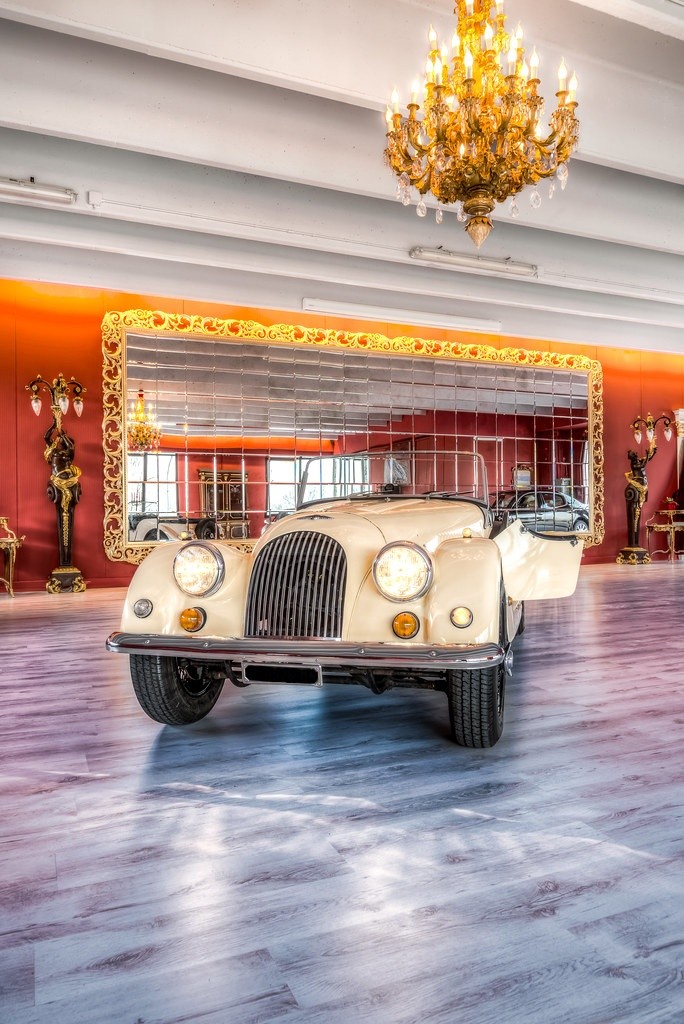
[518,496,527,508]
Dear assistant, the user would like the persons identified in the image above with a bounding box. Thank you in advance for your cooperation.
[44,415,75,478]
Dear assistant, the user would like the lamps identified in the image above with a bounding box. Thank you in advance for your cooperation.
[383,1,581,252]
[0,175,78,204]
[409,246,545,277]
[25,372,87,594]
[615,412,672,565]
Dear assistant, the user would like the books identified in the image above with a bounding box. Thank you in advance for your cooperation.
[628,447,657,485]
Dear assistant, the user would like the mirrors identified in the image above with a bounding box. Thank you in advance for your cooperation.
[127,383,163,457]
[100,308,605,565]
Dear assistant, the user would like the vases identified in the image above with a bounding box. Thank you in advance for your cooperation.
[667,501,675,510]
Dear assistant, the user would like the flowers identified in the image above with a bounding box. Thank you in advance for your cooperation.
[664,497,674,503]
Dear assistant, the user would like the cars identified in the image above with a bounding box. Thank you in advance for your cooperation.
[127,511,218,542]
[479,489,590,535]
[103,451,584,748]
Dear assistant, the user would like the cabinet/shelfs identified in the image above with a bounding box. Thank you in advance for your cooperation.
[198,469,251,540]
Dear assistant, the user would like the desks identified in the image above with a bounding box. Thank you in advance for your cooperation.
[645,510,684,562]
[0,516,26,598]
